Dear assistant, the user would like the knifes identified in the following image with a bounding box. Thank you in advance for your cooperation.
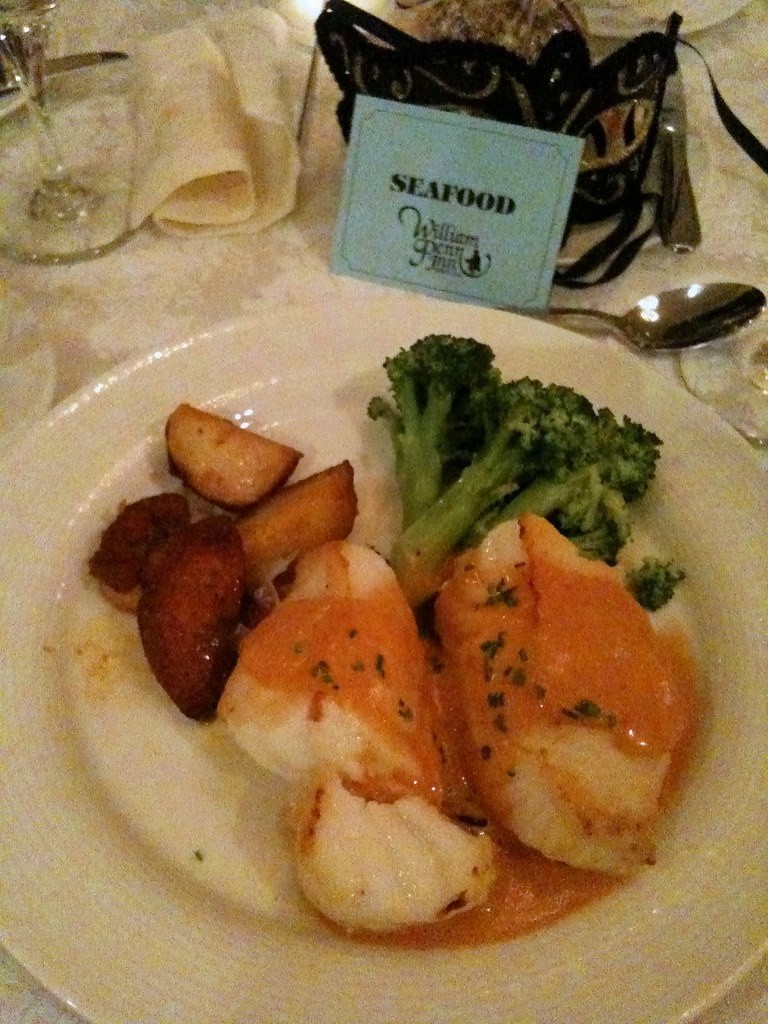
[0,50,128,90]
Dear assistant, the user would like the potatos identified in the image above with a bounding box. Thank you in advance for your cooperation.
[87,403,360,724]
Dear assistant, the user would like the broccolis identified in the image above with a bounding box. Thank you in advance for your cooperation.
[365,336,693,632]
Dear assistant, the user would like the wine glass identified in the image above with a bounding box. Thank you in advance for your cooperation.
[0,0,140,264]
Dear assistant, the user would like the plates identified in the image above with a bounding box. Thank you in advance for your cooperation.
[0,16,66,121]
[2,300,767,1022]
[585,0,753,37]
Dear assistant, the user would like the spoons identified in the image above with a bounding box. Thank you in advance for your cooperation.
[548,281,765,354]
